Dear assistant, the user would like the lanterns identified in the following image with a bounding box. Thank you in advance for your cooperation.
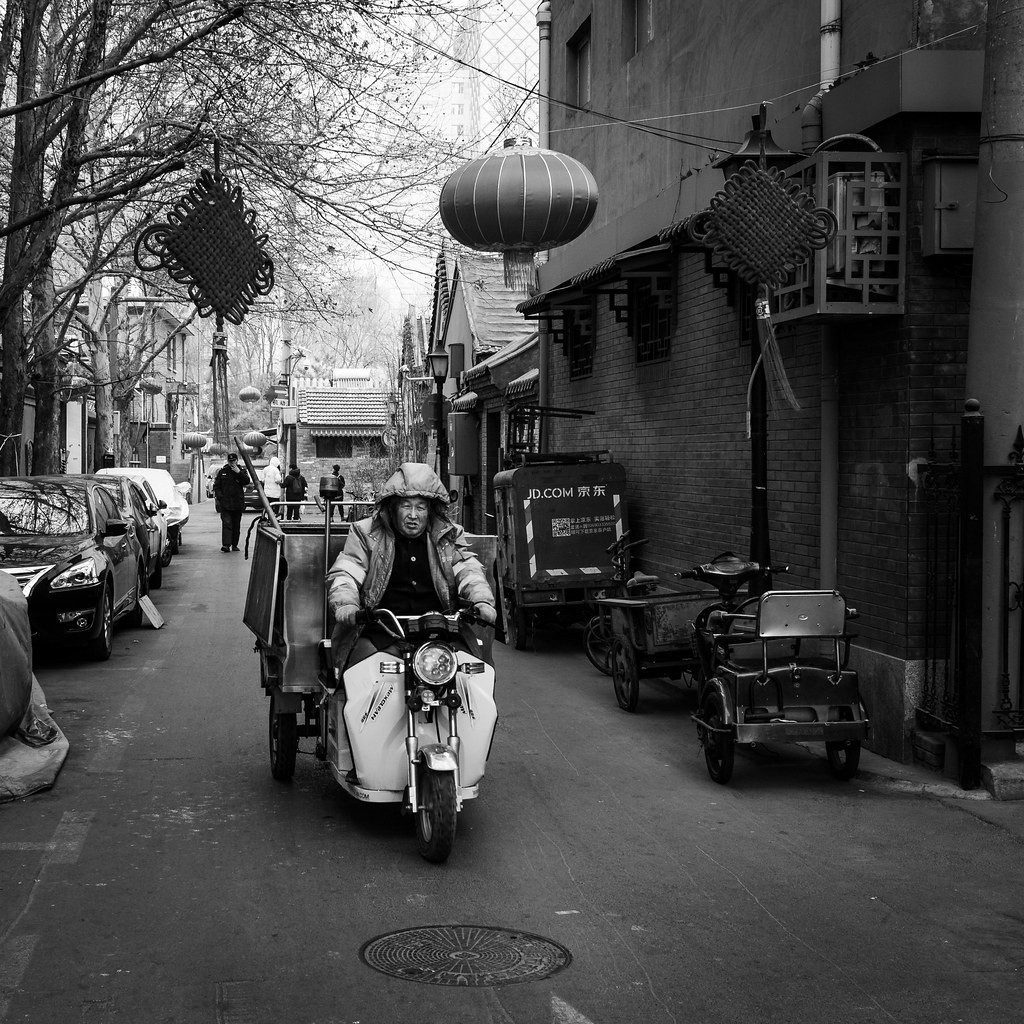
[65,377,93,397]
[243,432,267,446]
[182,433,206,448]
[438,134,600,260]
[139,377,163,395]
[210,444,226,455]
[238,386,261,402]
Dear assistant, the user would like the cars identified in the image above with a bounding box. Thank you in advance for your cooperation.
[204,463,224,498]
[1,476,147,663]
[97,467,191,557]
[131,477,174,565]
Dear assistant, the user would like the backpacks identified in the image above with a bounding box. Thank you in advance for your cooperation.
[288,474,304,494]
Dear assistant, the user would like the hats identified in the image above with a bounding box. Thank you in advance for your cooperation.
[227,453,238,463]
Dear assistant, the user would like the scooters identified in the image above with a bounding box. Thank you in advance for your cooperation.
[673,551,875,784]
[487,449,629,648]
[240,493,503,862]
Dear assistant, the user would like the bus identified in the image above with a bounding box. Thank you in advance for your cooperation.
[82,474,162,599]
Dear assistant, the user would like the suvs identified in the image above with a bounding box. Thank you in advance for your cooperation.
[234,461,278,511]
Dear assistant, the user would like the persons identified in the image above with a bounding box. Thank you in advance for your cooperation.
[330,465,347,523]
[213,454,250,553]
[262,457,283,515]
[280,463,308,520]
[324,462,499,785]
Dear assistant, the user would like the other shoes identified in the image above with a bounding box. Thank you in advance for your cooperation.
[221,545,240,552]
[344,767,360,784]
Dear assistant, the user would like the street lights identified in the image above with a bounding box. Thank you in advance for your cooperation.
[424,338,454,492]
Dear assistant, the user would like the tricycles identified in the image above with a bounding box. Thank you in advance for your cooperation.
[590,527,750,710]
